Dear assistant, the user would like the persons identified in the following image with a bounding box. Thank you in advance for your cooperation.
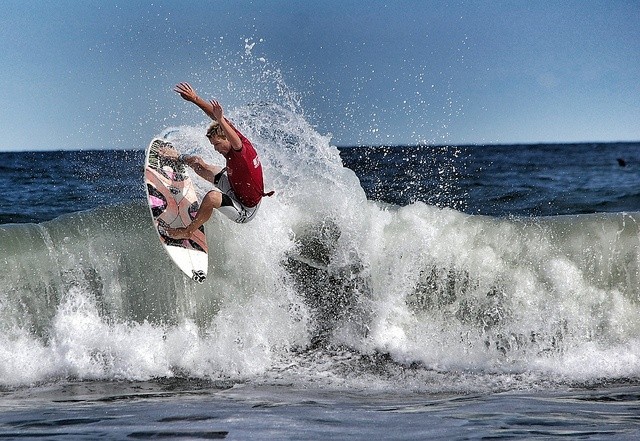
[157,82,264,241]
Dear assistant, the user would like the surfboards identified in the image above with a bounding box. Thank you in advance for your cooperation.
[144,138,208,285]
